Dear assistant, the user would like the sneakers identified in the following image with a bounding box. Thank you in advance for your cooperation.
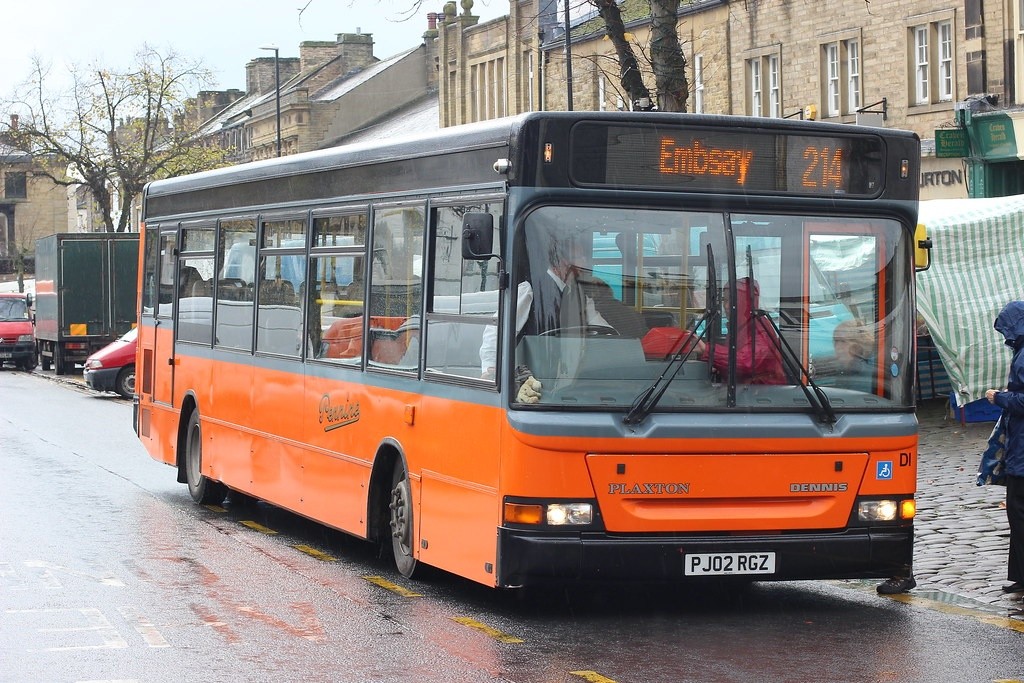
[876,577,916,594]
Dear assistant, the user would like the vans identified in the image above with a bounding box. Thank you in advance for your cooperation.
[81,322,141,396]
[1,292,38,373]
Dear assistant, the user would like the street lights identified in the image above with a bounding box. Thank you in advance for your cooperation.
[259,43,285,165]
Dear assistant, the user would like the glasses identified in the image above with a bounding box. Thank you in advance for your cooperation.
[573,255,586,261]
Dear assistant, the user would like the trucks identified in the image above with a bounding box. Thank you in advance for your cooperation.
[29,232,141,377]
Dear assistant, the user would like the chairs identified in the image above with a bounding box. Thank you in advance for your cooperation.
[192,278,338,314]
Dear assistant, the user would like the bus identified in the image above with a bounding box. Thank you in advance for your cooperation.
[131,107,924,588]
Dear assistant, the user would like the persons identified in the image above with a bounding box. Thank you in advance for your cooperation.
[479,228,620,380]
[684,277,786,385]
[985,301,1021,593]
[807,320,917,594]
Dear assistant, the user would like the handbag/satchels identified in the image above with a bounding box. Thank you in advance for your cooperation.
[975,407,1008,486]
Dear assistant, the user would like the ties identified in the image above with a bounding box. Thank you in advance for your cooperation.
[561,284,569,335]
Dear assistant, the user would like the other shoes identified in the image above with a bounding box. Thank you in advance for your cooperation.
[1002,582,1024,594]
